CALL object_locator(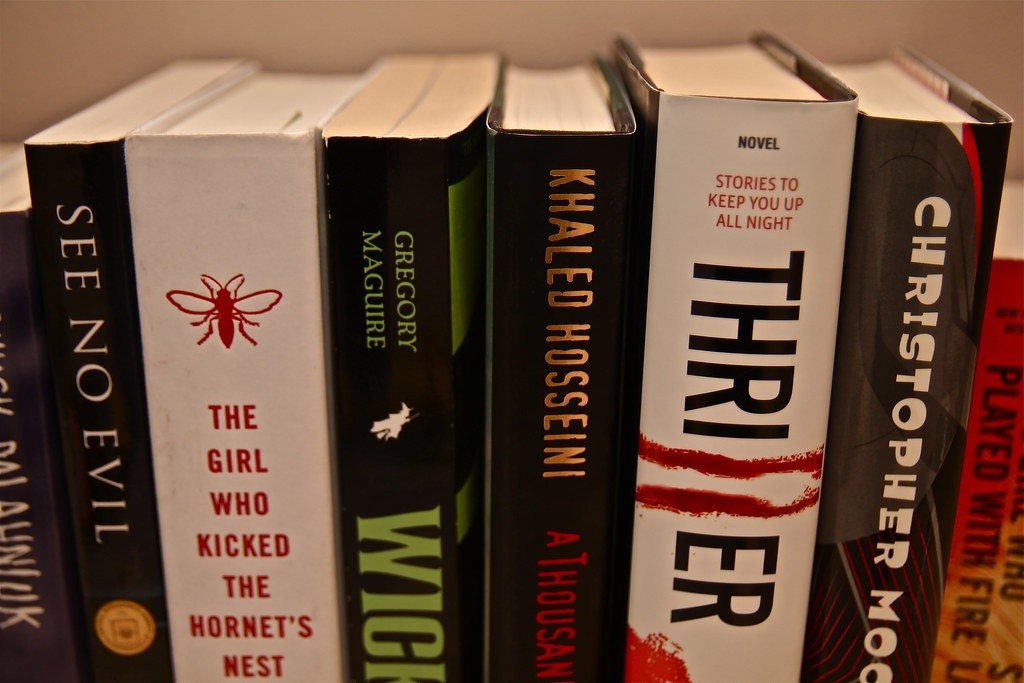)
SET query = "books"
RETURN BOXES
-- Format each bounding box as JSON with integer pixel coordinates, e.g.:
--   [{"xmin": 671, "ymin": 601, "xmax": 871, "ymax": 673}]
[
  {"xmin": 606, "ymin": 30, "xmax": 1012, "ymax": 683},
  {"xmin": 320, "ymin": 50, "xmax": 639, "ymax": 682},
  {"xmin": 0, "ymin": 58, "xmax": 363, "ymax": 683},
  {"xmin": 929, "ymin": 181, "xmax": 1024, "ymax": 683}
]
